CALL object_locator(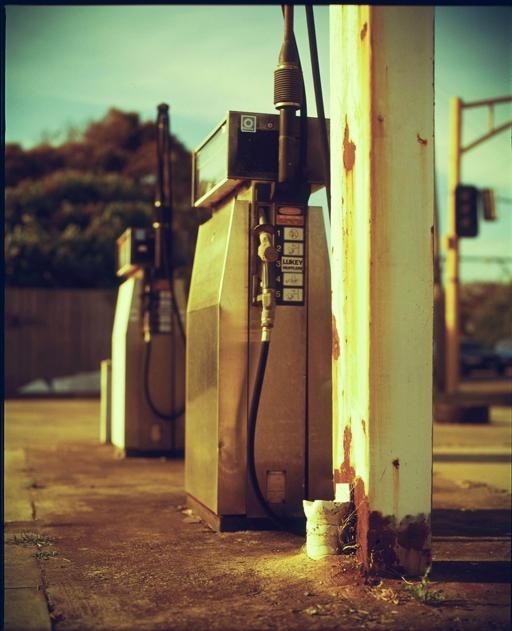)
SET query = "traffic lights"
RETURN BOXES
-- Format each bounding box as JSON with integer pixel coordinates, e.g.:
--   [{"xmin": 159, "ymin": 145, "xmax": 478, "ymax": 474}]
[{"xmin": 454, "ymin": 186, "xmax": 478, "ymax": 238}]
